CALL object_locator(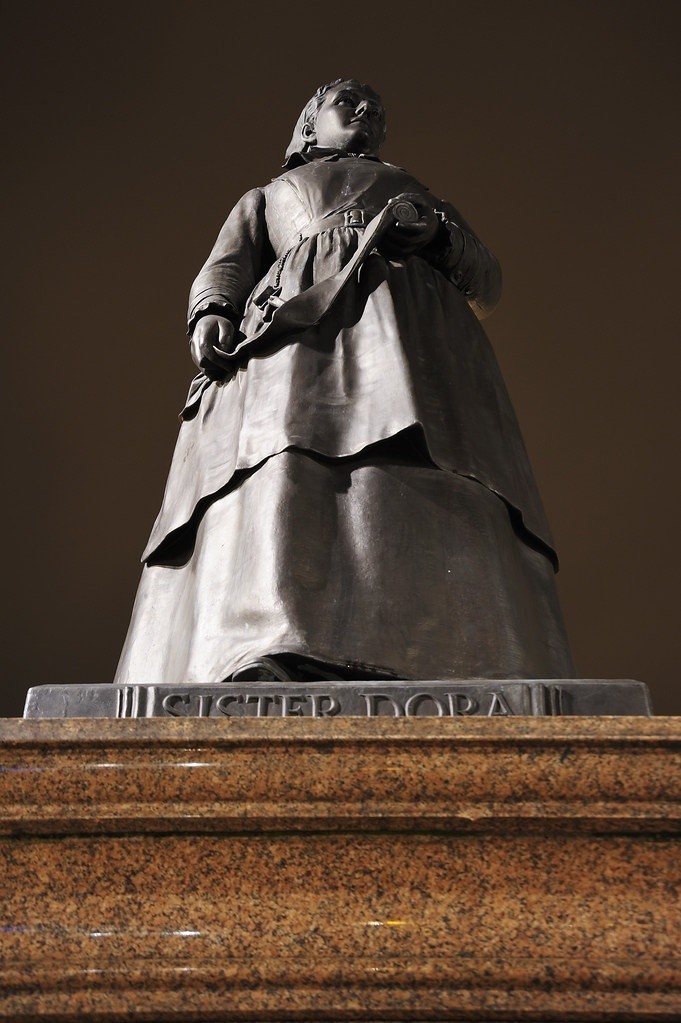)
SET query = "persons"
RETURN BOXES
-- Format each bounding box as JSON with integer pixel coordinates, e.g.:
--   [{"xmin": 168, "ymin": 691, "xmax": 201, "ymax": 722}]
[{"xmin": 114, "ymin": 74, "xmax": 581, "ymax": 681}]
